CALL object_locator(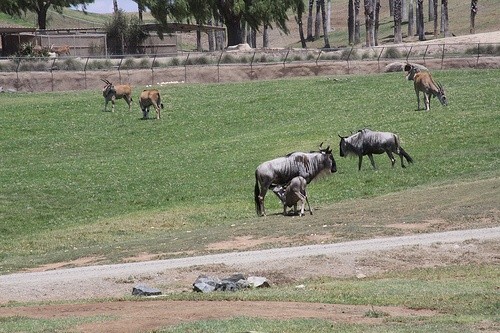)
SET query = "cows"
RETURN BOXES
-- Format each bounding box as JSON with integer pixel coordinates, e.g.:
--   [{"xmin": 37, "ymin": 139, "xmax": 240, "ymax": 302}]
[
  {"xmin": 100, "ymin": 78, "xmax": 133, "ymax": 113},
  {"xmin": 413, "ymin": 72, "xmax": 448, "ymax": 111},
  {"xmin": 254, "ymin": 142, "xmax": 337, "ymax": 217},
  {"xmin": 406, "ymin": 65, "xmax": 432, "ymax": 108},
  {"xmin": 338, "ymin": 128, "xmax": 413, "ymax": 169},
  {"xmin": 139, "ymin": 89, "xmax": 164, "ymax": 120},
  {"xmin": 32, "ymin": 39, "xmax": 71, "ymax": 57}
]
